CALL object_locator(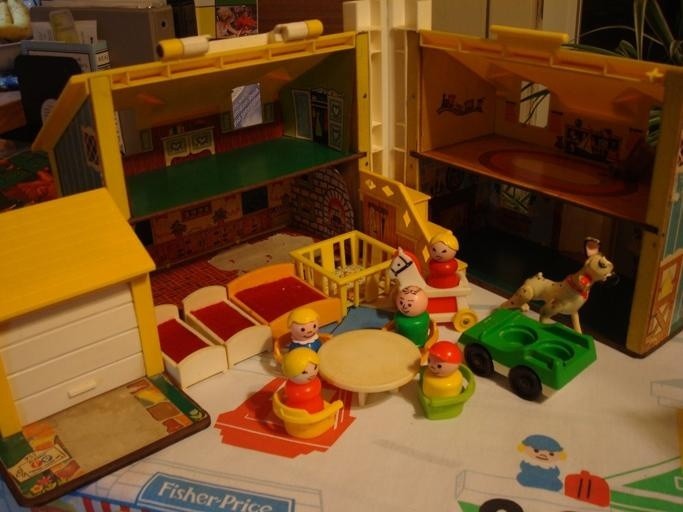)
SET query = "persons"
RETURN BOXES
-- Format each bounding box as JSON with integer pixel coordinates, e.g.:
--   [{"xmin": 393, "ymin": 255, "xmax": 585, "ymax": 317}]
[{"xmin": 214, "ymin": 5, "xmax": 255, "ymax": 38}]
